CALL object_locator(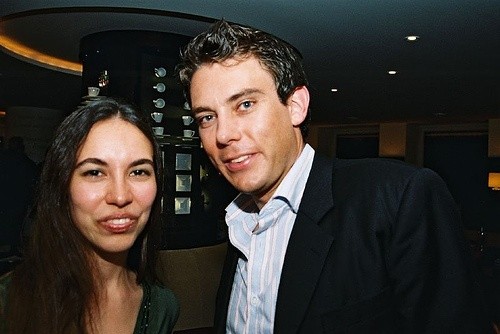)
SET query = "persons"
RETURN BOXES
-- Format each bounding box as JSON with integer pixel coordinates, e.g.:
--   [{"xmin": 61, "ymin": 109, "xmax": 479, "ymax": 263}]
[
  {"xmin": 175, "ymin": 21, "xmax": 489, "ymax": 334},
  {"xmin": 0, "ymin": 101, "xmax": 180, "ymax": 334}
]
